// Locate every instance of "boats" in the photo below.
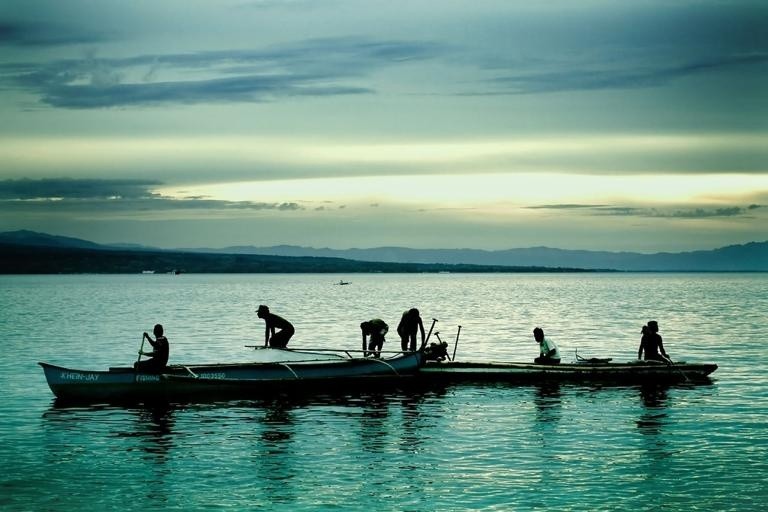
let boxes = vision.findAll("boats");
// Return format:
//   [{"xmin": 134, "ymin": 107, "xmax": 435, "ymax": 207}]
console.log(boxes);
[
  {"xmin": 335, "ymin": 279, "xmax": 353, "ymax": 287},
  {"xmin": 28, "ymin": 348, "xmax": 433, "ymax": 400},
  {"xmin": 422, "ymin": 359, "xmax": 722, "ymax": 385}
]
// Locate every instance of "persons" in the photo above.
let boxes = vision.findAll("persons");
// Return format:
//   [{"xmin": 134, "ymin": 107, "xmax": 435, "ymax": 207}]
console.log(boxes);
[
  {"xmin": 134, "ymin": 323, "xmax": 169, "ymax": 372},
  {"xmin": 396, "ymin": 307, "xmax": 425, "ymax": 353},
  {"xmin": 533, "ymin": 327, "xmax": 562, "ymax": 363},
  {"xmin": 254, "ymin": 304, "xmax": 295, "ymax": 348},
  {"xmin": 638, "ymin": 320, "xmax": 669, "ymax": 362},
  {"xmin": 360, "ymin": 318, "xmax": 389, "ymax": 358}
]
